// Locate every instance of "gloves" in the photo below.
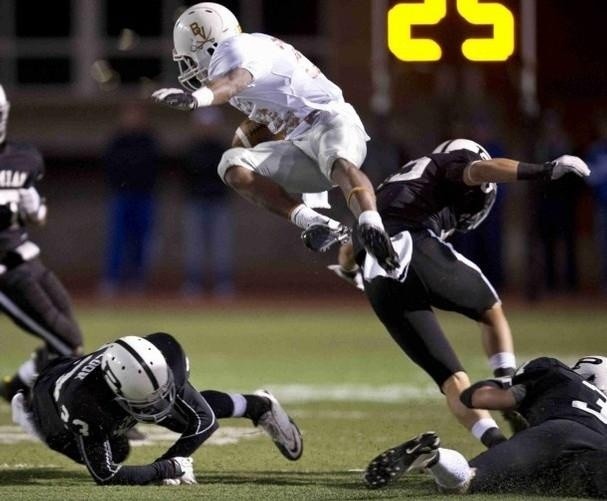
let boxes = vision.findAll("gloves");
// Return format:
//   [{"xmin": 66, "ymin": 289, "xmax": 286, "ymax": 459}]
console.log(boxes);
[
  {"xmin": 327, "ymin": 265, "xmax": 367, "ymax": 291},
  {"xmin": 16, "ymin": 186, "xmax": 49, "ymax": 225},
  {"xmin": 149, "ymin": 86, "xmax": 215, "ymax": 112},
  {"xmin": 516, "ymin": 154, "xmax": 592, "ymax": 186}
]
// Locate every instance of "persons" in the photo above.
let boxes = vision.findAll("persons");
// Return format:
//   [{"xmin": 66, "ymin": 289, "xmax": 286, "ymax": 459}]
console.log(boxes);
[
  {"xmin": 327, "ymin": 139, "xmax": 591, "ymax": 448},
  {"xmin": 364, "ymin": 357, "xmax": 607, "ymax": 500},
  {"xmin": 523, "ymin": 109, "xmax": 580, "ymax": 292},
  {"xmin": 12, "ymin": 333, "xmax": 302, "ymax": 484},
  {"xmin": 103, "ymin": 97, "xmax": 175, "ymax": 296},
  {"xmin": 173, "ymin": 106, "xmax": 238, "ymax": 299},
  {"xmin": 0, "ymin": 84, "xmax": 82, "ymax": 403},
  {"xmin": 154, "ymin": 3, "xmax": 400, "ymax": 278}
]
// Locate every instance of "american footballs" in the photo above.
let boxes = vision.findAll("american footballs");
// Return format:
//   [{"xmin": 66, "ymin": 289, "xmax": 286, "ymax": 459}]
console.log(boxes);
[{"xmin": 232, "ymin": 119, "xmax": 285, "ymax": 149}]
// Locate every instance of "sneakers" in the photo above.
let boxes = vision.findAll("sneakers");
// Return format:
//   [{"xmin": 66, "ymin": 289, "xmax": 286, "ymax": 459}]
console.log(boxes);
[
  {"xmin": 357, "ymin": 224, "xmax": 401, "ymax": 276},
  {"xmin": 249, "ymin": 389, "xmax": 304, "ymax": 463},
  {"xmin": 300, "ymin": 222, "xmax": 354, "ymax": 254},
  {"xmin": 362, "ymin": 431, "xmax": 441, "ymax": 490}
]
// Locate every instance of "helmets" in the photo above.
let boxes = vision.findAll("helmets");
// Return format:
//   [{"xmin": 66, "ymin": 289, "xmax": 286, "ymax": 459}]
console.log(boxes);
[
  {"xmin": 570, "ymin": 357, "xmax": 607, "ymax": 397},
  {"xmin": 172, "ymin": 2, "xmax": 240, "ymax": 92},
  {"xmin": 100, "ymin": 334, "xmax": 176, "ymax": 426},
  {"xmin": 431, "ymin": 138, "xmax": 494, "ymax": 160}
]
